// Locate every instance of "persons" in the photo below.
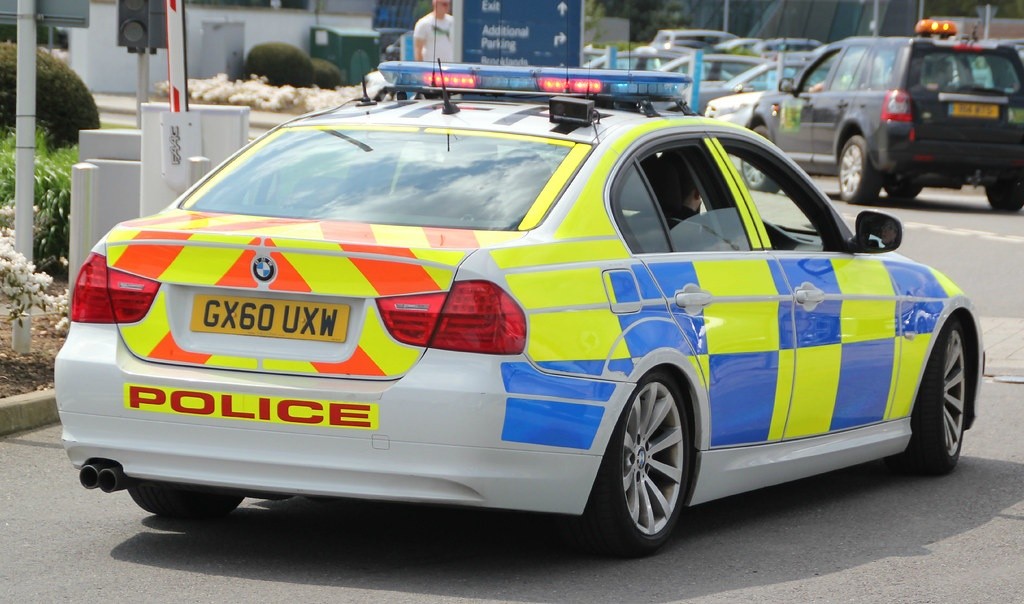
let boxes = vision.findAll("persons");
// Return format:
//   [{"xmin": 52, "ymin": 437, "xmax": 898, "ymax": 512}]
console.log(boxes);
[
  {"xmin": 880, "ymin": 220, "xmax": 899, "ymax": 247},
  {"xmin": 414, "ymin": 0, "xmax": 455, "ymax": 63},
  {"xmin": 652, "ymin": 151, "xmax": 712, "ymax": 231},
  {"xmin": 808, "ymin": 80, "xmax": 826, "ymax": 92}
]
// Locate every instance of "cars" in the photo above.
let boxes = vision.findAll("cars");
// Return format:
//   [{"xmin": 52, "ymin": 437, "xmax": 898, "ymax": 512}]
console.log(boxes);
[
  {"xmin": 580, "ymin": 26, "xmax": 1024, "ymax": 129},
  {"xmin": 51, "ymin": 57, "xmax": 988, "ymax": 560}
]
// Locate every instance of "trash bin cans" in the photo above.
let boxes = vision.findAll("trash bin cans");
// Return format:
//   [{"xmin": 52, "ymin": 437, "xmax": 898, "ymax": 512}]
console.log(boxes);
[
  {"xmin": 310, "ymin": 25, "xmax": 383, "ymax": 88},
  {"xmin": 372, "ymin": 26, "xmax": 408, "ymax": 65}
]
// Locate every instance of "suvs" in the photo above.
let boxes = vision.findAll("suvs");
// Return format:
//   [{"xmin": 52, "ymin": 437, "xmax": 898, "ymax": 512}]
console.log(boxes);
[{"xmin": 741, "ymin": 18, "xmax": 1024, "ymax": 213}]
